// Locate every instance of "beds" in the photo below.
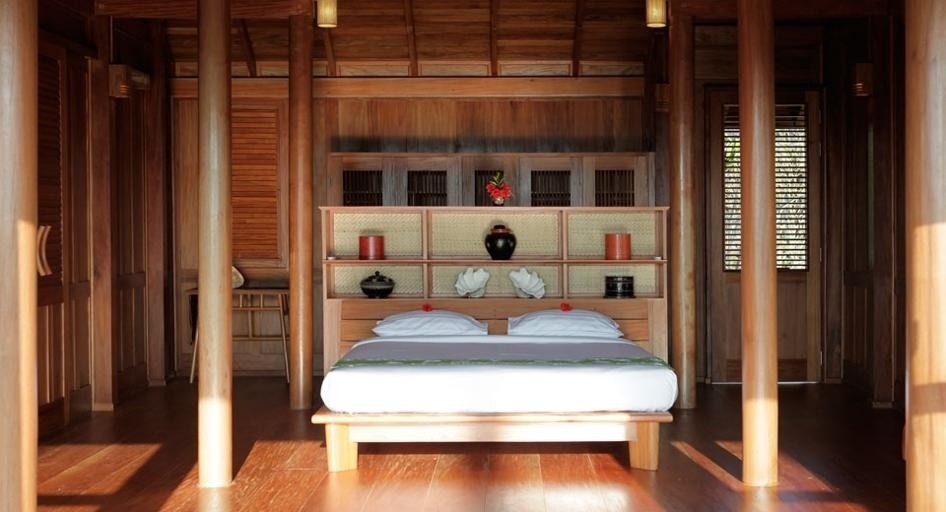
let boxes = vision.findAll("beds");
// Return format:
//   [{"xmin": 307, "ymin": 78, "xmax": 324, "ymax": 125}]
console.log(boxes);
[{"xmin": 311, "ymin": 299, "xmax": 678, "ymax": 472}]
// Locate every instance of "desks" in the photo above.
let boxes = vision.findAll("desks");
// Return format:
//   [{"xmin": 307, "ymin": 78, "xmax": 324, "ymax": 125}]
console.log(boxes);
[{"xmin": 184, "ymin": 287, "xmax": 290, "ymax": 385}]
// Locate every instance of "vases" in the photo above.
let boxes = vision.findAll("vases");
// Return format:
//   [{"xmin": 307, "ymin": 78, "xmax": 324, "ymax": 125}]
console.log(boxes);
[{"xmin": 493, "ymin": 197, "xmax": 505, "ymax": 206}]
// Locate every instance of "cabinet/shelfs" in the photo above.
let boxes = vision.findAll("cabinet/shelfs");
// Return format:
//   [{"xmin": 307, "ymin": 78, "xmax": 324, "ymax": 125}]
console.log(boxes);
[
  {"xmin": 317, "ymin": 205, "xmax": 671, "ymax": 378},
  {"xmin": 326, "ymin": 152, "xmax": 655, "ymax": 206}
]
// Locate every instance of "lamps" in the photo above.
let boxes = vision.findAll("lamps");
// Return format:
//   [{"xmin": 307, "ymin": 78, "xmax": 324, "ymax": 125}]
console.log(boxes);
[
  {"xmin": 850, "ymin": 63, "xmax": 875, "ymax": 97},
  {"xmin": 317, "ymin": 0, "xmax": 338, "ymax": 28},
  {"xmin": 645, "ymin": 0, "xmax": 666, "ymax": 28},
  {"xmin": 655, "ymin": 31, "xmax": 671, "ymax": 112},
  {"xmin": 108, "ymin": 64, "xmax": 132, "ymax": 98}
]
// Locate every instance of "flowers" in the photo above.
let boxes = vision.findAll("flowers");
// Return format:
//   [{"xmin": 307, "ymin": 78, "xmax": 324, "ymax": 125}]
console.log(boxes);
[{"xmin": 486, "ymin": 172, "xmax": 512, "ymax": 200}]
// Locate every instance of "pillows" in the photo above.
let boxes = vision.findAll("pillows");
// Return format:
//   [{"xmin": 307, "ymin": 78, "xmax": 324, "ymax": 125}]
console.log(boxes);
[
  {"xmin": 371, "ymin": 304, "xmax": 488, "ymax": 337},
  {"xmin": 507, "ymin": 303, "xmax": 624, "ymax": 338}
]
